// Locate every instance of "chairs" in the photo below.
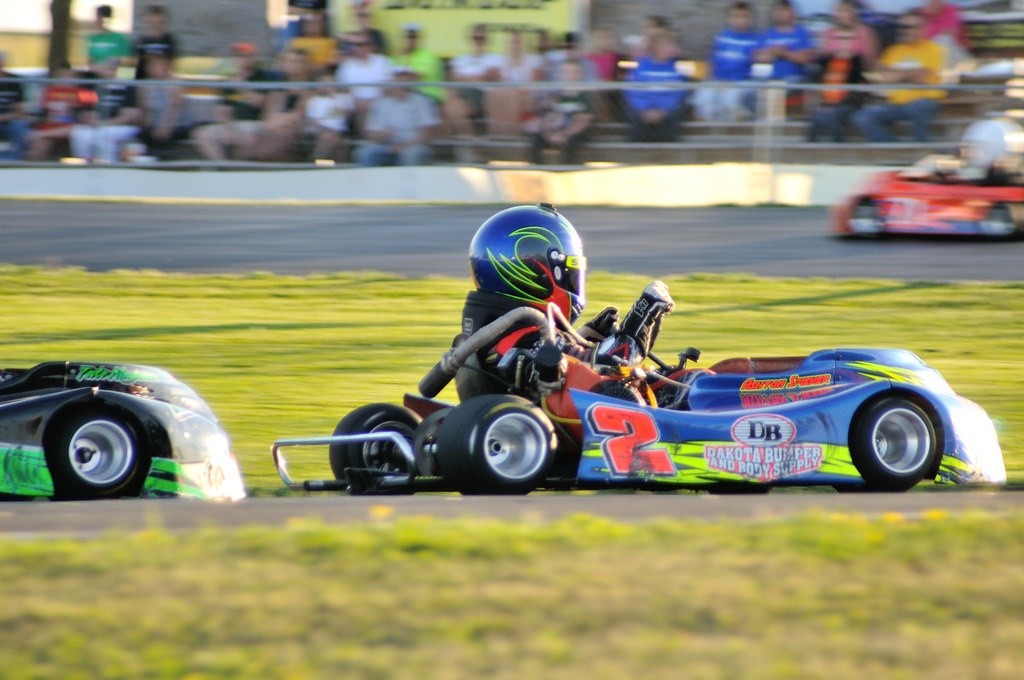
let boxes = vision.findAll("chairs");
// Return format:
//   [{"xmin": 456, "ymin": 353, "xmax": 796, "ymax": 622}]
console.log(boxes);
[{"xmin": 451, "ymin": 334, "xmax": 582, "ymax": 471}]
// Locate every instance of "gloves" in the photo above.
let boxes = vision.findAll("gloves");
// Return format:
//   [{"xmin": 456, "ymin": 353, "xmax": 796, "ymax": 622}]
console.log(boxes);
[
  {"xmin": 587, "ymin": 308, "xmax": 617, "ymax": 342},
  {"xmin": 645, "ymin": 281, "xmax": 674, "ymax": 314}
]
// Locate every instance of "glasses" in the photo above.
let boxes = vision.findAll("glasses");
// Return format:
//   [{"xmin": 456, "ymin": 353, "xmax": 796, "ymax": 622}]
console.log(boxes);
[{"xmin": 348, "ymin": 41, "xmax": 373, "ymax": 48}]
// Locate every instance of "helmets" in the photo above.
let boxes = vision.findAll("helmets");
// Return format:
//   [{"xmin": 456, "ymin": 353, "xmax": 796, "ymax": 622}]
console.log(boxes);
[
  {"xmin": 961, "ymin": 118, "xmax": 1024, "ymax": 185},
  {"xmin": 469, "ymin": 204, "xmax": 585, "ymax": 332}
]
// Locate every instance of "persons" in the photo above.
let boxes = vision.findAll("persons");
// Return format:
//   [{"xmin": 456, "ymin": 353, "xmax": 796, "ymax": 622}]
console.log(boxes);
[
  {"xmin": 938, "ymin": 117, "xmax": 1024, "ymax": 186},
  {"xmin": 460, "ymin": 200, "xmax": 676, "ymax": 403},
  {"xmin": 0, "ymin": 0, "xmax": 973, "ymax": 165}
]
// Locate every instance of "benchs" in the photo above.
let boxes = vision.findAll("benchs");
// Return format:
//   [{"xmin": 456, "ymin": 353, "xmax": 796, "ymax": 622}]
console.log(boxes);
[{"xmin": 179, "ymin": 71, "xmax": 1024, "ymax": 152}]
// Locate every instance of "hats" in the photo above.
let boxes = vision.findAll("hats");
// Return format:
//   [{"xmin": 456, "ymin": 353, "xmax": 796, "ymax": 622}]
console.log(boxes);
[
  {"xmin": 391, "ymin": 66, "xmax": 421, "ymax": 79},
  {"xmin": 230, "ymin": 42, "xmax": 256, "ymax": 58}
]
